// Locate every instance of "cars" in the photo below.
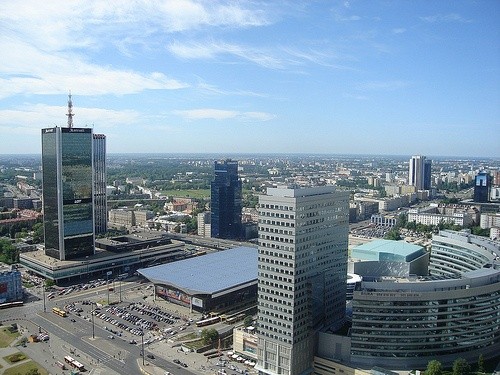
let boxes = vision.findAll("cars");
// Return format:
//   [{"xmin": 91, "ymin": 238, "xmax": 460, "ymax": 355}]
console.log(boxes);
[
  {"xmin": 47, "ymin": 272, "xmax": 137, "ymax": 300},
  {"xmin": 180, "ymin": 362, "xmax": 187, "ymax": 367},
  {"xmin": 174, "ymin": 359, "xmax": 180, "ymax": 363},
  {"xmin": 92, "ymin": 302, "xmax": 193, "ymax": 346},
  {"xmin": 39, "ymin": 333, "xmax": 49, "ymax": 341},
  {"xmin": 148, "ymin": 354, "xmax": 154, "ymax": 359}
]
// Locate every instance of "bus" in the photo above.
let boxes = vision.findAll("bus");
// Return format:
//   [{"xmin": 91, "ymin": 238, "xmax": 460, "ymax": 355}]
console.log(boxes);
[
  {"xmin": 196, "ymin": 316, "xmax": 220, "ymax": 327},
  {"xmin": 53, "ymin": 306, "xmax": 66, "ymax": 317},
  {"xmin": 64, "ymin": 355, "xmax": 84, "ymax": 371},
  {"xmin": 226, "ymin": 313, "xmax": 246, "ymax": 325}
]
